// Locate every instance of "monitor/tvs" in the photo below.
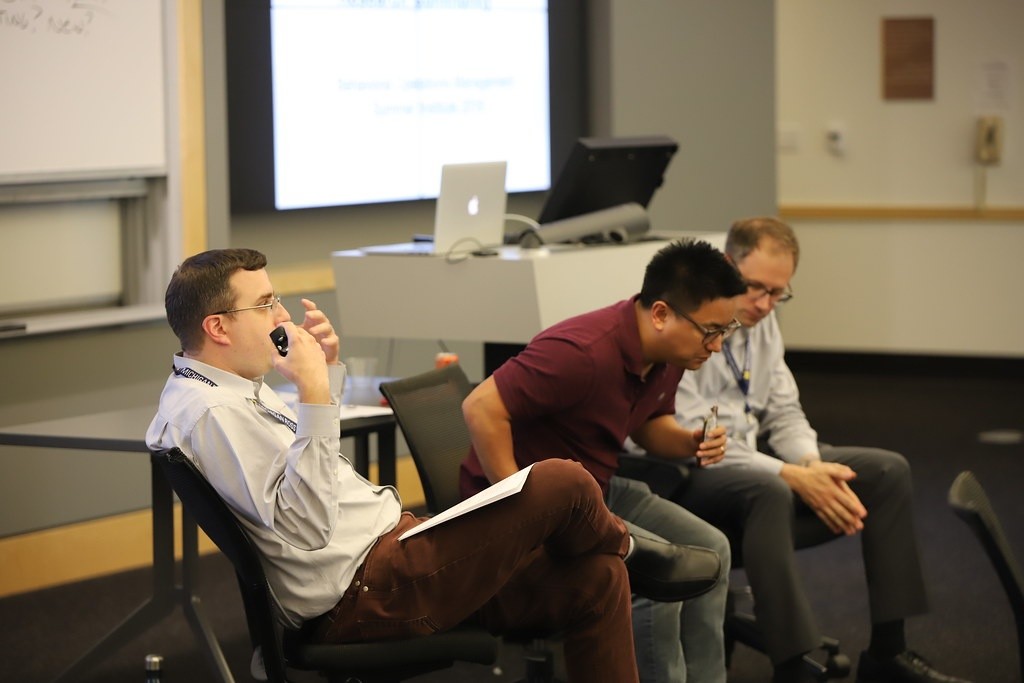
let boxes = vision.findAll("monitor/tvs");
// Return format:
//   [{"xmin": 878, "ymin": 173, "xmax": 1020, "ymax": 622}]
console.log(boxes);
[{"xmin": 536, "ymin": 134, "xmax": 678, "ymax": 225}]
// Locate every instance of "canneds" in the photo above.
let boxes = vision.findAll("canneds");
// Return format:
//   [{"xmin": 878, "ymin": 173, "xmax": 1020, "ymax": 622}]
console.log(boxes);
[{"xmin": 435, "ymin": 352, "xmax": 459, "ymax": 368}]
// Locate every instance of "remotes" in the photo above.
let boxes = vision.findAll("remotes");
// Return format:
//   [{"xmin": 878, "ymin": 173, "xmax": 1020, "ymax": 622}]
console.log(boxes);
[{"xmin": 269, "ymin": 325, "xmax": 289, "ymax": 357}]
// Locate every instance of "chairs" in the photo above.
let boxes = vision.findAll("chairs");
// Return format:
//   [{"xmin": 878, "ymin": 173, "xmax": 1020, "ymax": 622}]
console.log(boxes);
[
  {"xmin": 149, "ymin": 447, "xmax": 501, "ymax": 683},
  {"xmin": 378, "ymin": 366, "xmax": 850, "ymax": 683},
  {"xmin": 948, "ymin": 470, "xmax": 1024, "ymax": 677}
]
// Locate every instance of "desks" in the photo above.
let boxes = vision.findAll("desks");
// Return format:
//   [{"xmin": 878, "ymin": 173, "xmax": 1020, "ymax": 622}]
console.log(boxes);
[
  {"xmin": 0, "ymin": 392, "xmax": 399, "ymax": 683},
  {"xmin": 333, "ymin": 232, "xmax": 729, "ymax": 380}
]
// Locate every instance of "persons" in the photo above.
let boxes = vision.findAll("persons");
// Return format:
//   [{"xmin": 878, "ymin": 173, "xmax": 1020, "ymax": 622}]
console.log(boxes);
[
  {"xmin": 142, "ymin": 248, "xmax": 721, "ymax": 683},
  {"xmin": 625, "ymin": 218, "xmax": 968, "ymax": 683},
  {"xmin": 456, "ymin": 239, "xmax": 749, "ymax": 683}
]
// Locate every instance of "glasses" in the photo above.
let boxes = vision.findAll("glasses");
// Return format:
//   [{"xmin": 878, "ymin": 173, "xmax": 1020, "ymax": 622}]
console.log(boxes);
[
  {"xmin": 729, "ymin": 254, "xmax": 793, "ymax": 302},
  {"xmin": 673, "ymin": 303, "xmax": 742, "ymax": 347},
  {"xmin": 210, "ymin": 293, "xmax": 280, "ymax": 314}
]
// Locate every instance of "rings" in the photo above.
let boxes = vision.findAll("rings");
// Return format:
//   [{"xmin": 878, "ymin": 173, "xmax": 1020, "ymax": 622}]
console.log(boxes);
[{"xmin": 721, "ymin": 446, "xmax": 725, "ymax": 453}]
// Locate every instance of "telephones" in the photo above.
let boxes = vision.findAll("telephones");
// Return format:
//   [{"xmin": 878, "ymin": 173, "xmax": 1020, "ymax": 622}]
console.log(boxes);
[{"xmin": 975, "ymin": 117, "xmax": 1003, "ymax": 163}]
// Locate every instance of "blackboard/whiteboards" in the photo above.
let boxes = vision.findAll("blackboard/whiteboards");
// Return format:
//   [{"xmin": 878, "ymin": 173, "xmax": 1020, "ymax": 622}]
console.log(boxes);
[{"xmin": 0, "ymin": 0, "xmax": 168, "ymax": 184}]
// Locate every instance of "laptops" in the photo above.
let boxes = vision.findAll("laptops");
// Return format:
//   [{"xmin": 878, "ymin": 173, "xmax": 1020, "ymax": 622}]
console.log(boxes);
[{"xmin": 363, "ymin": 160, "xmax": 507, "ymax": 257}]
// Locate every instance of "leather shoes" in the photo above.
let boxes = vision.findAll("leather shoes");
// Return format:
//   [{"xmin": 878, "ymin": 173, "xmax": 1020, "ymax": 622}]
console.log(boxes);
[
  {"xmin": 856, "ymin": 646, "xmax": 967, "ymax": 683},
  {"xmin": 626, "ymin": 531, "xmax": 722, "ymax": 604},
  {"xmin": 772, "ymin": 654, "xmax": 828, "ymax": 683}
]
